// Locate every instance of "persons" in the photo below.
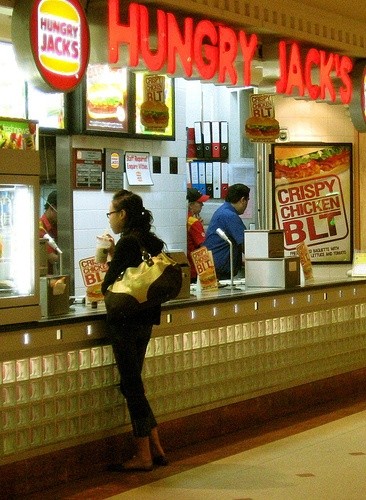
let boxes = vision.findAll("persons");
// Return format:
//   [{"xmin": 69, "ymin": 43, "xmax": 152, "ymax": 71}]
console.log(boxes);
[
  {"xmin": 187, "ymin": 187, "xmax": 209, "ymax": 282},
  {"xmin": 201, "ymin": 183, "xmax": 250, "ymax": 280},
  {"xmin": 100, "ymin": 190, "xmax": 181, "ymax": 471},
  {"xmin": 39, "ymin": 191, "xmax": 57, "ymax": 273}
]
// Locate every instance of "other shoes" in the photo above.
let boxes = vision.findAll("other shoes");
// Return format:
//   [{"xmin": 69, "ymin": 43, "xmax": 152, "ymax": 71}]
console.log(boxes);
[{"xmin": 108, "ymin": 455, "xmax": 169, "ymax": 473}]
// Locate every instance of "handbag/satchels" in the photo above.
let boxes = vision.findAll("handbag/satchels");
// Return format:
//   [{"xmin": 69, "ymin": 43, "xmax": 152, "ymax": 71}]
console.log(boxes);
[{"xmin": 104, "ymin": 234, "xmax": 183, "ymax": 324}]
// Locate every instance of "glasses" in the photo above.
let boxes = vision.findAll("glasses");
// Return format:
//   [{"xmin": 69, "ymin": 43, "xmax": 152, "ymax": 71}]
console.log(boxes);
[{"xmin": 106, "ymin": 210, "xmax": 119, "ymax": 218}]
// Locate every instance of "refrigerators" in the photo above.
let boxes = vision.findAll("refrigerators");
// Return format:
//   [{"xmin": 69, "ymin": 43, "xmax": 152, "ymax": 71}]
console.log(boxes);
[{"xmin": 2, "ymin": 113, "xmax": 41, "ymax": 327}]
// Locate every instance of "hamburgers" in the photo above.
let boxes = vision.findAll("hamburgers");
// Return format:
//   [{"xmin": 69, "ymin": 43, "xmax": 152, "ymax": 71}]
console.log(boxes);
[
  {"xmin": 275, "ymin": 144, "xmax": 350, "ymax": 184},
  {"xmin": 88, "ymin": 88, "xmax": 168, "ymax": 130},
  {"xmin": 87, "ymin": 284, "xmax": 102, "ymax": 301},
  {"xmin": 200, "ymin": 270, "xmax": 216, "ymax": 288},
  {"xmin": 245, "ymin": 117, "xmax": 280, "ymax": 142}
]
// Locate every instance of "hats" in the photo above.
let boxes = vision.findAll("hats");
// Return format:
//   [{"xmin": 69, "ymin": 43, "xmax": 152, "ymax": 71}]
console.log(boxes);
[{"xmin": 186, "ymin": 187, "xmax": 210, "ymax": 203}]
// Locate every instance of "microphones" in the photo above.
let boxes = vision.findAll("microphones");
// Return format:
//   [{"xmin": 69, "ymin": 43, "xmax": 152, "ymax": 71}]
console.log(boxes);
[
  {"xmin": 42, "ymin": 234, "xmax": 62, "ymax": 275},
  {"xmin": 216, "ymin": 227, "xmax": 241, "ymax": 290}
]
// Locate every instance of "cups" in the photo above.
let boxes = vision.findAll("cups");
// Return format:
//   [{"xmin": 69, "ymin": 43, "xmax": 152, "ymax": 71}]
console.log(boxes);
[{"xmin": 94, "ymin": 235, "xmax": 112, "ymax": 264}]
